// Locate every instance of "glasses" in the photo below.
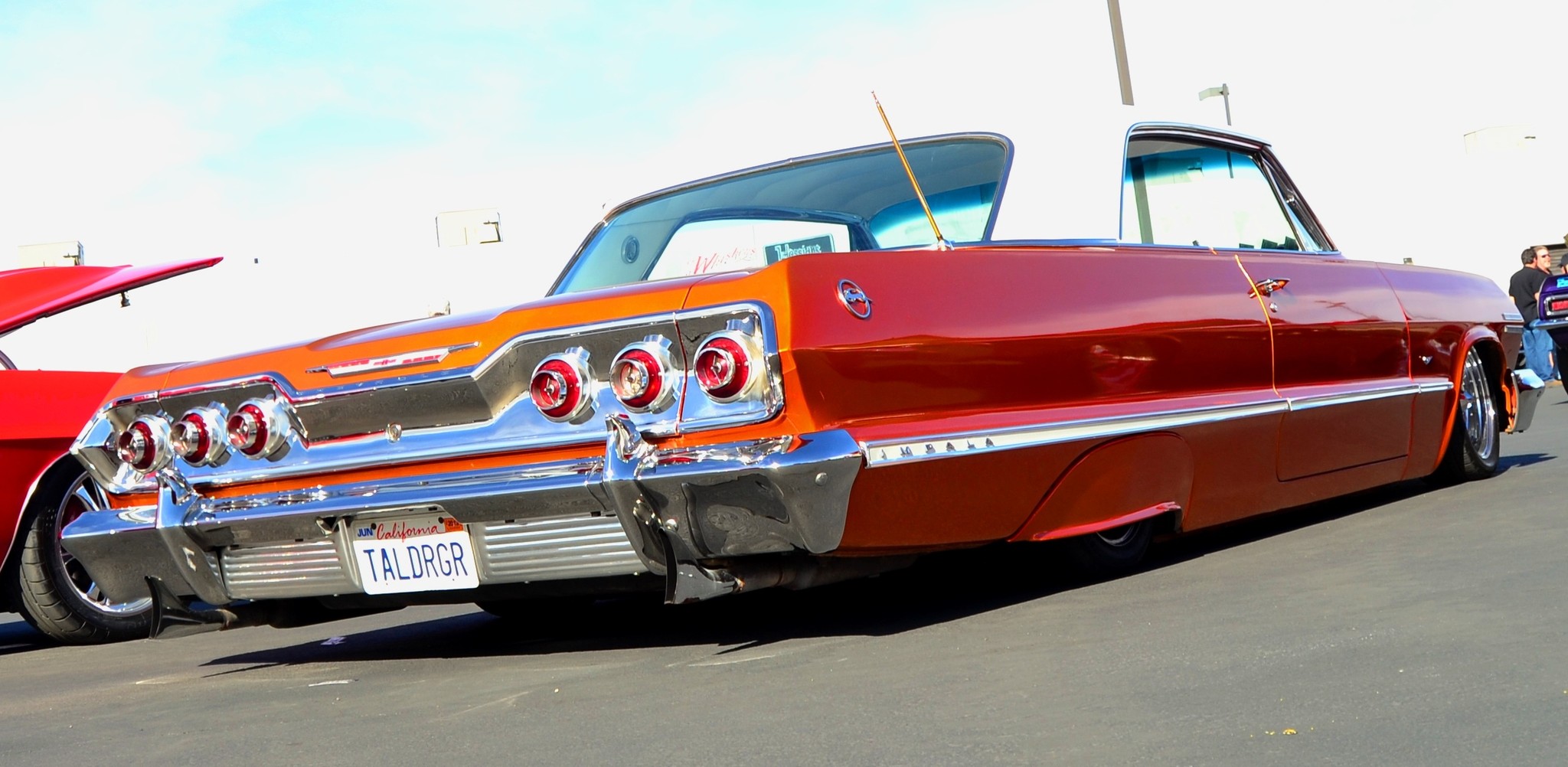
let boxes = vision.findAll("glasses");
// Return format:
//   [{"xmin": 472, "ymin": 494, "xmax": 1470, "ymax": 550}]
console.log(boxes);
[
  {"xmin": 1560, "ymin": 265, "xmax": 1563, "ymax": 268},
  {"xmin": 1542, "ymin": 254, "xmax": 1550, "ymax": 258}
]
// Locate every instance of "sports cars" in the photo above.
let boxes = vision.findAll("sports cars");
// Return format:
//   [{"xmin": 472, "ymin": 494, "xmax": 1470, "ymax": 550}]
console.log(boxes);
[
  {"xmin": 63, "ymin": 89, "xmax": 1546, "ymax": 632},
  {"xmin": 0, "ymin": 257, "xmax": 228, "ymax": 650}
]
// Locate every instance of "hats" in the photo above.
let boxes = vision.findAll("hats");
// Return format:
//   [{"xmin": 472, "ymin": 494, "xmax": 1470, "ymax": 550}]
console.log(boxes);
[{"xmin": 1557, "ymin": 253, "xmax": 1568, "ymax": 266}]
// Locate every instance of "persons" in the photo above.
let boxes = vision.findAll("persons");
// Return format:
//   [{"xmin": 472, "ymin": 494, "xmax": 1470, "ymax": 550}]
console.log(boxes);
[
  {"xmin": 1508, "ymin": 248, "xmax": 1565, "ymax": 387},
  {"xmin": 1558, "ymin": 251, "xmax": 1568, "ymax": 282},
  {"xmin": 1529, "ymin": 245, "xmax": 1560, "ymax": 381}
]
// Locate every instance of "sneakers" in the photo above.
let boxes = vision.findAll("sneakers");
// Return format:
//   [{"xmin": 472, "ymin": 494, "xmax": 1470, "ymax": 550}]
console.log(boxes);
[{"xmin": 1545, "ymin": 379, "xmax": 1562, "ymax": 387}]
